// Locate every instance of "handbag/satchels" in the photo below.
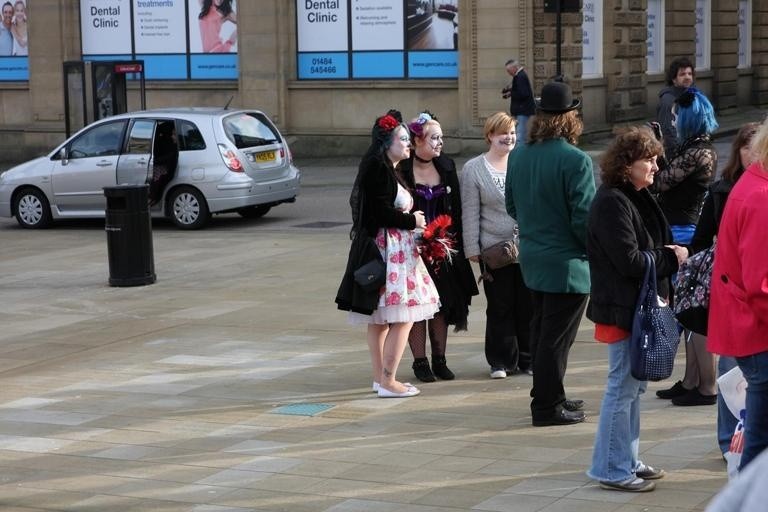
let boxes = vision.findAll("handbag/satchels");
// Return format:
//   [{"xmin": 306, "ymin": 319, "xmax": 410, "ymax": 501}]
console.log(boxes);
[{"xmin": 629, "ymin": 249, "xmax": 680, "ymax": 382}]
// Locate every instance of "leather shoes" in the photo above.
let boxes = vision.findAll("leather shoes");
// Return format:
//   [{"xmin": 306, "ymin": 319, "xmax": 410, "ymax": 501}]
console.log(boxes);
[
  {"xmin": 373, "ymin": 381, "xmax": 415, "ymax": 394},
  {"xmin": 532, "ymin": 406, "xmax": 586, "ymax": 427},
  {"xmin": 562, "ymin": 400, "xmax": 586, "ymax": 411},
  {"xmin": 672, "ymin": 386, "xmax": 718, "ymax": 407},
  {"xmin": 655, "ymin": 379, "xmax": 693, "ymax": 400},
  {"xmin": 378, "ymin": 387, "xmax": 421, "ymax": 399}
]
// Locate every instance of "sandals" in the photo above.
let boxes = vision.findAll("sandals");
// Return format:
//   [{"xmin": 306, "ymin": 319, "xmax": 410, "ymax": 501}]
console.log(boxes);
[
  {"xmin": 636, "ymin": 462, "xmax": 666, "ymax": 480},
  {"xmin": 600, "ymin": 473, "xmax": 656, "ymax": 492}
]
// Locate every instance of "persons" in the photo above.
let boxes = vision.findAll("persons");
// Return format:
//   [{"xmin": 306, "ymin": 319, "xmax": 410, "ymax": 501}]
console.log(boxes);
[
  {"xmin": 708, "ymin": 117, "xmax": 766, "ymax": 476},
  {"xmin": 335, "ymin": 110, "xmax": 442, "ymax": 398},
  {"xmin": 147, "ymin": 130, "xmax": 184, "ymax": 205},
  {"xmin": 9, "ymin": 1, "xmax": 27, "ymax": 56},
  {"xmin": 658, "ymin": 60, "xmax": 702, "ymax": 151},
  {"xmin": 651, "ymin": 94, "xmax": 718, "ymax": 406},
  {"xmin": 198, "ymin": 0, "xmax": 236, "ymax": 53},
  {"xmin": 0, "ymin": 2, "xmax": 13, "ymax": 56},
  {"xmin": 587, "ymin": 124, "xmax": 688, "ymax": 494},
  {"xmin": 504, "ymin": 60, "xmax": 537, "ymax": 146},
  {"xmin": 504, "ymin": 82, "xmax": 596, "ymax": 425},
  {"xmin": 459, "ymin": 114, "xmax": 532, "ymax": 377},
  {"xmin": 666, "ymin": 123, "xmax": 759, "ymax": 461},
  {"xmin": 397, "ymin": 112, "xmax": 479, "ymax": 381}
]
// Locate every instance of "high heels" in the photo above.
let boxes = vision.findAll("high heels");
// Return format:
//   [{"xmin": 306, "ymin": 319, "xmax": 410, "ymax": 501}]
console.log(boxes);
[
  {"xmin": 412, "ymin": 356, "xmax": 437, "ymax": 383},
  {"xmin": 430, "ymin": 354, "xmax": 456, "ymax": 381}
]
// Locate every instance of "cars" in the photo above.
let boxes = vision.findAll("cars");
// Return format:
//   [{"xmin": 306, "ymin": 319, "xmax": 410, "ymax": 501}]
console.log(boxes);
[{"xmin": 0, "ymin": 96, "xmax": 301, "ymax": 229}]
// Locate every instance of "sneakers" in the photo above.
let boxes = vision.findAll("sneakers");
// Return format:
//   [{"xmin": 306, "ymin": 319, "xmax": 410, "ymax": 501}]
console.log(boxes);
[
  {"xmin": 524, "ymin": 364, "xmax": 534, "ymax": 375},
  {"xmin": 489, "ymin": 365, "xmax": 508, "ymax": 379}
]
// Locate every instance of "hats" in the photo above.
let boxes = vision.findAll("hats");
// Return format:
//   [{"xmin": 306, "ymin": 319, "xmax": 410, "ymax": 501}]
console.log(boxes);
[{"xmin": 535, "ymin": 83, "xmax": 581, "ymax": 115}]
